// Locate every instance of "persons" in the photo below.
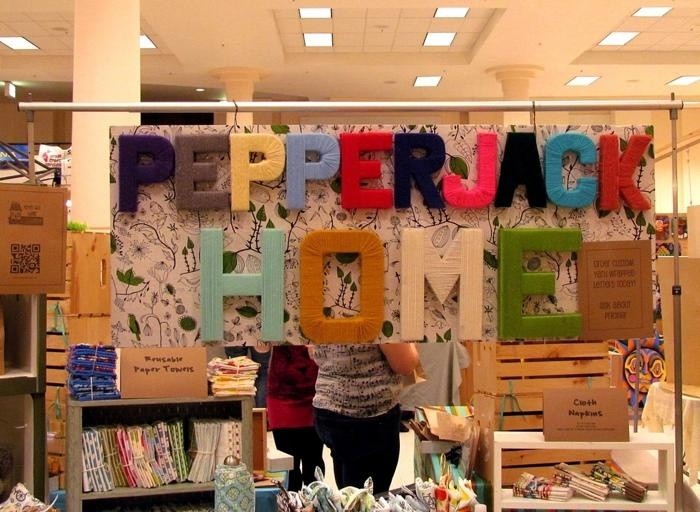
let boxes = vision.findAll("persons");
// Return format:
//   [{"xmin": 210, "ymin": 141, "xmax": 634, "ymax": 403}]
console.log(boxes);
[
  {"xmin": 305, "ymin": 343, "xmax": 420, "ymax": 494},
  {"xmin": 266, "ymin": 342, "xmax": 325, "ymax": 493}
]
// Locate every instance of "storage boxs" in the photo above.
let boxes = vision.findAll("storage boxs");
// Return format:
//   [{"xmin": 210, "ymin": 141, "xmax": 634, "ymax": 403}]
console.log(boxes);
[
  {"xmin": 472, "ymin": 392, "xmax": 613, "ymax": 490},
  {"xmin": 472, "ymin": 339, "xmax": 611, "ymax": 397},
  {"xmin": 41, "ymin": 227, "xmax": 117, "ymax": 491}
]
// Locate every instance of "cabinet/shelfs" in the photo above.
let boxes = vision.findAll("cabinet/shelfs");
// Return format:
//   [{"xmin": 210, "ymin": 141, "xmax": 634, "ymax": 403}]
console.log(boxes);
[
  {"xmin": 0, "ymin": 293, "xmax": 45, "ymax": 512},
  {"xmin": 65, "ymin": 395, "xmax": 254, "ymax": 512},
  {"xmin": 494, "ymin": 432, "xmax": 674, "ymax": 512}
]
